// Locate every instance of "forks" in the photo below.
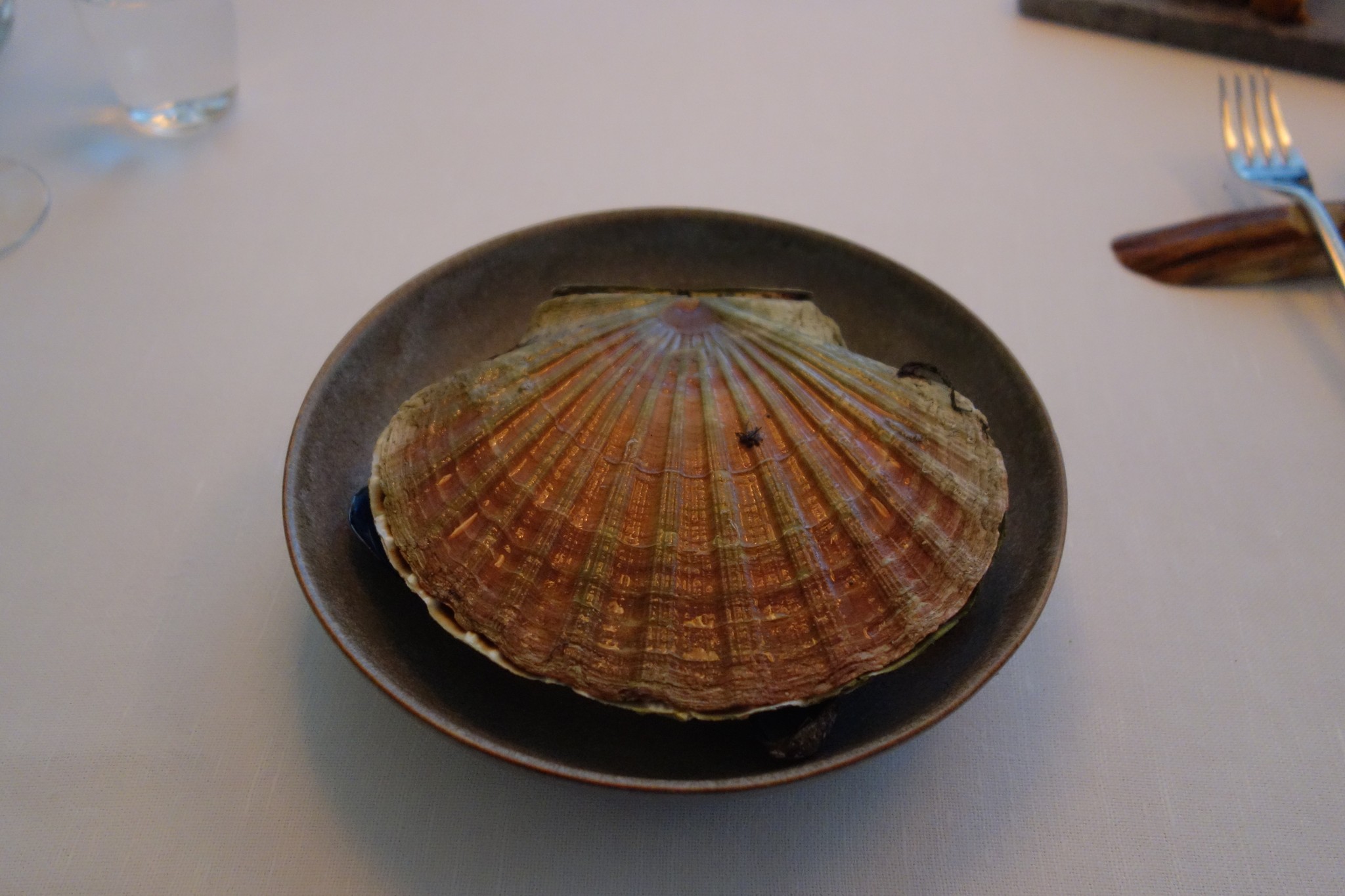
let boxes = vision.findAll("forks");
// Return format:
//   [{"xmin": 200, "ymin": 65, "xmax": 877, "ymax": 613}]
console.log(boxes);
[{"xmin": 1218, "ymin": 72, "xmax": 1345, "ymax": 296}]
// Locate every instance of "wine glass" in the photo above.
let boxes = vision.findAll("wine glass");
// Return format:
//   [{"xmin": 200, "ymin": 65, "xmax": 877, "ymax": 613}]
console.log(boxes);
[{"xmin": 2, "ymin": 0, "xmax": 49, "ymax": 256}]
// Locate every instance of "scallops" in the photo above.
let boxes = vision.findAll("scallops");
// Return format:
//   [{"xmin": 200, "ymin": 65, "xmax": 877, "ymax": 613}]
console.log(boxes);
[{"xmin": 370, "ymin": 293, "xmax": 1009, "ymax": 722}]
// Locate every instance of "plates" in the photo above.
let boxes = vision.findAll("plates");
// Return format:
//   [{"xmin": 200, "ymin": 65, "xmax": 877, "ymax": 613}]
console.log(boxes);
[{"xmin": 283, "ymin": 205, "xmax": 1068, "ymax": 792}]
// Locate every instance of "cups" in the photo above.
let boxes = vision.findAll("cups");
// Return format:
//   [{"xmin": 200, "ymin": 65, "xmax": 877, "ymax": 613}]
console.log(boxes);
[{"xmin": 75, "ymin": 0, "xmax": 239, "ymax": 135}]
